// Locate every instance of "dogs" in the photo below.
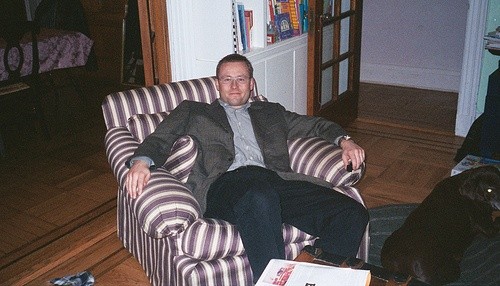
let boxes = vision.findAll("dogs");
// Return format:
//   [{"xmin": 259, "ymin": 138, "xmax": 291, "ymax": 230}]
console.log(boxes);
[{"xmin": 379, "ymin": 163, "xmax": 500, "ymax": 286}]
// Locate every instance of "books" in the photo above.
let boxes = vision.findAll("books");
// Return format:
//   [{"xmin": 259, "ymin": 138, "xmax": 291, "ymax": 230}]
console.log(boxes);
[
  {"xmin": 232, "ymin": 0, "xmax": 254, "ymax": 55},
  {"xmin": 451, "ymin": 154, "xmax": 500, "ymax": 177},
  {"xmin": 255, "ymin": 259, "xmax": 371, "ymax": 286}
]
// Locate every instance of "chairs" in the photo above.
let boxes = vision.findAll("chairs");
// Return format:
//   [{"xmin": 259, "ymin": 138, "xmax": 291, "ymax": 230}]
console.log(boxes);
[{"xmin": 0, "ymin": 0, "xmax": 89, "ymax": 159}]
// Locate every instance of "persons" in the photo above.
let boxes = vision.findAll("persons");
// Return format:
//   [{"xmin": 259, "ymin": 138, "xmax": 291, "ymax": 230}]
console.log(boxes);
[{"xmin": 124, "ymin": 54, "xmax": 370, "ymax": 284}]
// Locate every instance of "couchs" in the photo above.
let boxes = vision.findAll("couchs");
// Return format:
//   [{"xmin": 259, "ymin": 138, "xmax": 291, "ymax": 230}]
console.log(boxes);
[{"xmin": 102, "ymin": 77, "xmax": 369, "ymax": 286}]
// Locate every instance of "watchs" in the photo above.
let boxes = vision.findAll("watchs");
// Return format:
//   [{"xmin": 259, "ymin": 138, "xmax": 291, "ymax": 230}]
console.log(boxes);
[{"xmin": 338, "ymin": 134, "xmax": 352, "ymax": 148}]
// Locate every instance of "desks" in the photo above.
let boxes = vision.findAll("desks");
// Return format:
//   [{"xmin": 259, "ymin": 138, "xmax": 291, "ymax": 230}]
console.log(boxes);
[{"xmin": 0, "ymin": 28, "xmax": 95, "ymax": 80}]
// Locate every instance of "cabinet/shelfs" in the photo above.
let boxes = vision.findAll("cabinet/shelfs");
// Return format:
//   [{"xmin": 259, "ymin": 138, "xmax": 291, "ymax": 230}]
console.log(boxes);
[{"xmin": 252, "ymin": 34, "xmax": 333, "ymax": 116}]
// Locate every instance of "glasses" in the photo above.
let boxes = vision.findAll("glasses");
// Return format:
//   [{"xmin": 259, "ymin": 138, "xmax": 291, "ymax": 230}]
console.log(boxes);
[{"xmin": 219, "ymin": 77, "xmax": 247, "ymax": 84}]
